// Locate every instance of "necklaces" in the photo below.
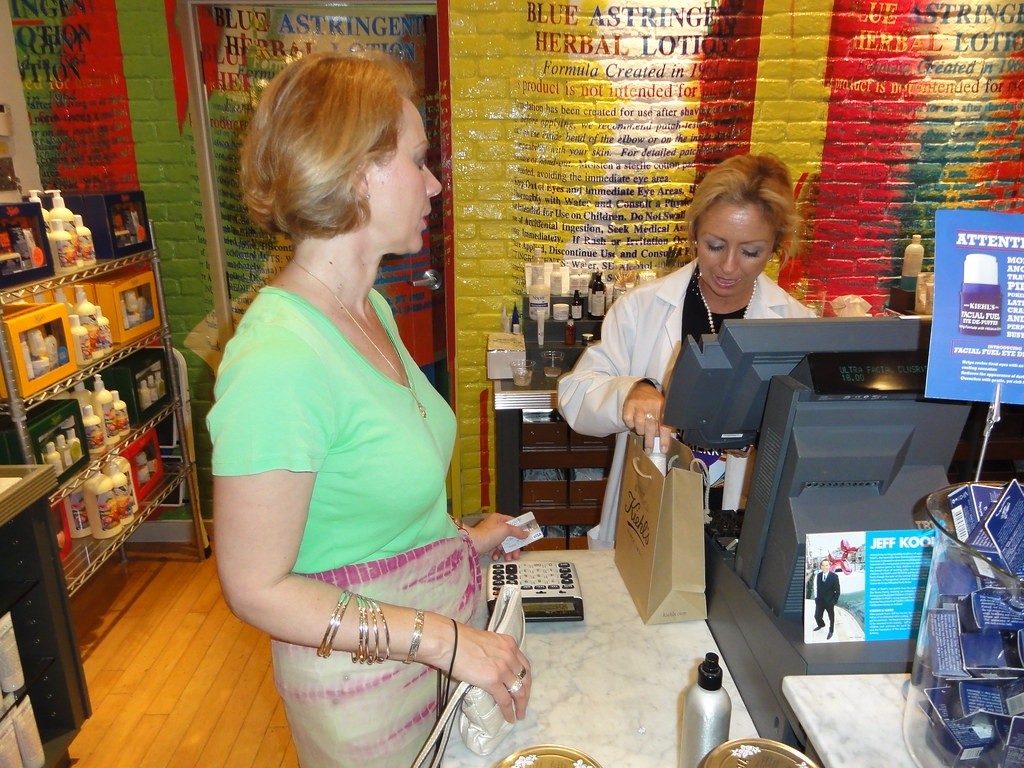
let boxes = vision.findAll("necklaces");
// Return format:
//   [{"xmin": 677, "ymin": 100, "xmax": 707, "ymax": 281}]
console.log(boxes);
[
  {"xmin": 294, "ymin": 255, "xmax": 428, "ymax": 418},
  {"xmin": 696, "ymin": 274, "xmax": 758, "ymax": 335}
]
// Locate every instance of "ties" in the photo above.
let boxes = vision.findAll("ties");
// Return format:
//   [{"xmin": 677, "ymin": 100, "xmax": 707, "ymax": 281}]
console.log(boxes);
[{"xmin": 824, "ymin": 576, "xmax": 826, "ymax": 580}]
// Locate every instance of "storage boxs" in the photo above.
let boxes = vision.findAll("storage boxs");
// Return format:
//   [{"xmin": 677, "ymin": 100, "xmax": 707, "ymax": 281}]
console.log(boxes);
[
  {"xmin": 85, "ymin": 349, "xmax": 173, "ymax": 425},
  {"xmin": 0, "ymin": 301, "xmax": 77, "ymax": 398},
  {"xmin": 95, "ymin": 269, "xmax": 161, "ymax": 344},
  {"xmin": 0, "ymin": 203, "xmax": 54, "ymax": 287},
  {"xmin": 84, "ymin": 192, "xmax": 153, "ymax": 259},
  {"xmin": 487, "ymin": 333, "xmax": 526, "ymax": 379},
  {"xmin": 2, "ymin": 400, "xmax": 91, "ymax": 486},
  {"xmin": 116, "ymin": 428, "xmax": 164, "ymax": 502}
]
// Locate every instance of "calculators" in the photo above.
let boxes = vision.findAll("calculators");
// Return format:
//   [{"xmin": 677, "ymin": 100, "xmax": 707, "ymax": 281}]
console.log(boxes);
[{"xmin": 486, "ymin": 561, "xmax": 583, "ymax": 622}]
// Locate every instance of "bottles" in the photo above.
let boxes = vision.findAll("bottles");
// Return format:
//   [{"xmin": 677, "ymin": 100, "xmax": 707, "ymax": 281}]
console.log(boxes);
[
  {"xmin": 94, "ymin": 305, "xmax": 114, "ymax": 354},
  {"xmin": 47, "ymin": 219, "xmax": 78, "ymax": 274},
  {"xmin": 613, "ymin": 283, "xmax": 626, "ymax": 304},
  {"xmin": 900, "ymin": 234, "xmax": 924, "ymax": 289},
  {"xmin": 532, "ymin": 247, "xmax": 546, "ymax": 286},
  {"xmin": 69, "ymin": 315, "xmax": 93, "ymax": 365},
  {"xmin": 586, "ymin": 270, "xmax": 606, "ymax": 319},
  {"xmin": 43, "ymin": 428, "xmax": 84, "ymax": 476},
  {"xmin": 74, "ymin": 215, "xmax": 96, "ymax": 265},
  {"xmin": 645, "ymin": 436, "xmax": 666, "ymax": 478},
  {"xmin": 554, "ymin": 304, "xmax": 570, "ymax": 322},
  {"xmin": 529, "ymin": 278, "xmax": 550, "ymax": 320},
  {"xmin": 140, "ymin": 371, "xmax": 167, "ymax": 409},
  {"xmin": 119, "ymin": 288, "xmax": 155, "ymax": 329},
  {"xmin": 82, "ymin": 405, "xmax": 106, "ymax": 452},
  {"xmin": 111, "ymin": 390, "xmax": 131, "ymax": 436},
  {"xmin": 679, "ymin": 652, "xmax": 732, "ymax": 768},
  {"xmin": 550, "ymin": 263, "xmax": 562, "ymax": 296},
  {"xmin": 19, "ymin": 322, "xmax": 69, "ymax": 380}
]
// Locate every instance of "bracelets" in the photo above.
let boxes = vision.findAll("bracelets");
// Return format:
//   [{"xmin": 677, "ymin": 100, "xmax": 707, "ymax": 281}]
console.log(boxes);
[{"xmin": 318, "ymin": 589, "xmax": 424, "ymax": 663}]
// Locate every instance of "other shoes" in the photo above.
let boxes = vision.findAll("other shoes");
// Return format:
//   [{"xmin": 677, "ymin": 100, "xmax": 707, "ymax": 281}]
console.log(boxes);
[
  {"xmin": 814, "ymin": 622, "xmax": 826, "ymax": 633},
  {"xmin": 830, "ymin": 629, "xmax": 835, "ymax": 641}
]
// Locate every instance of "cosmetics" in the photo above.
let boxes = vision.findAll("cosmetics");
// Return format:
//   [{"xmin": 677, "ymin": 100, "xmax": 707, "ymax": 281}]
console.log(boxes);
[{"xmin": 0, "ymin": 610, "xmax": 45, "ymax": 767}]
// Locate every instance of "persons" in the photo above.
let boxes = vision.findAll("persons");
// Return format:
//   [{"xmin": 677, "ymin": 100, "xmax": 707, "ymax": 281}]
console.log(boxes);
[
  {"xmin": 558, "ymin": 154, "xmax": 818, "ymax": 549},
  {"xmin": 205, "ymin": 57, "xmax": 532, "ymax": 765},
  {"xmin": 814, "ymin": 560, "xmax": 840, "ymax": 640}
]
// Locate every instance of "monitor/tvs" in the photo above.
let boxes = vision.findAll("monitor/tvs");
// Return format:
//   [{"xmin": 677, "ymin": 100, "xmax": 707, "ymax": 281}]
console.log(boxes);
[{"xmin": 661, "ymin": 312, "xmax": 940, "ymax": 449}]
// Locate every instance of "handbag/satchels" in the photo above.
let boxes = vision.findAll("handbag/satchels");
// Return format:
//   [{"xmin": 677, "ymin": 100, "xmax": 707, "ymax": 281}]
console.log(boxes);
[
  {"xmin": 412, "ymin": 581, "xmax": 528, "ymax": 768},
  {"xmin": 615, "ymin": 438, "xmax": 707, "ymax": 625}
]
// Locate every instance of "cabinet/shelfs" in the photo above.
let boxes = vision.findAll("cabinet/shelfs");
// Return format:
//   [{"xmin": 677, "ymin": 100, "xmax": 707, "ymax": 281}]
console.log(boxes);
[
  {"xmin": 0, "ymin": 466, "xmax": 92, "ymax": 768},
  {"xmin": 0, "ymin": 217, "xmax": 204, "ymax": 583},
  {"xmin": 491, "ymin": 370, "xmax": 619, "ymax": 555}
]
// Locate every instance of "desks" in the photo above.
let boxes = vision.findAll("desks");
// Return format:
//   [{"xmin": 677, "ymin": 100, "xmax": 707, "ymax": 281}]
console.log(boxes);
[{"xmin": 438, "ymin": 549, "xmax": 761, "ymax": 768}]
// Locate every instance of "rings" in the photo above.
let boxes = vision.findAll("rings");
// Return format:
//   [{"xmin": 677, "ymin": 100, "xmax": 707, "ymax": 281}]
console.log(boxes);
[
  {"xmin": 509, "ymin": 677, "xmax": 523, "ymax": 693},
  {"xmin": 519, "ymin": 665, "xmax": 526, "ymax": 678},
  {"xmin": 646, "ymin": 413, "xmax": 656, "ymax": 421}
]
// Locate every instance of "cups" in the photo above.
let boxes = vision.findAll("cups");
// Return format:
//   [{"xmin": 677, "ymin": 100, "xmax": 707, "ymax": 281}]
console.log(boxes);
[
  {"xmin": 789, "ymin": 282, "xmax": 826, "ymax": 318},
  {"xmin": 510, "ymin": 360, "xmax": 535, "ymax": 385},
  {"xmin": 541, "ymin": 350, "xmax": 565, "ymax": 377}
]
